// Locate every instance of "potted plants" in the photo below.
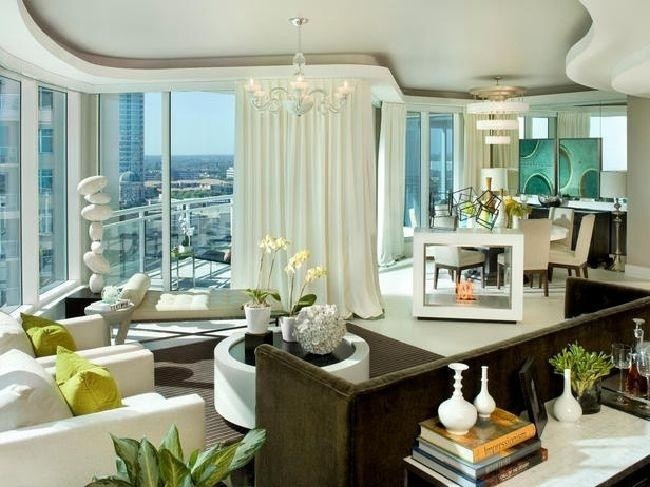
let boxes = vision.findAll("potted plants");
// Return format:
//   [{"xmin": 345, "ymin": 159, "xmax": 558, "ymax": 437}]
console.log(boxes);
[
  {"xmin": 242, "ymin": 234, "xmax": 328, "ymax": 343},
  {"xmin": 461, "ymin": 199, "xmax": 532, "ymax": 230},
  {"xmin": 548, "ymin": 340, "xmax": 616, "ymax": 415}
]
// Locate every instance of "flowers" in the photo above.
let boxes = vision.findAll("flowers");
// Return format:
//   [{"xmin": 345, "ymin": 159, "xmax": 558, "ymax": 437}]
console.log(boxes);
[
  {"xmin": 176, "ymin": 205, "xmax": 193, "ymax": 246},
  {"xmin": 292, "ymin": 303, "xmax": 347, "ymax": 355}
]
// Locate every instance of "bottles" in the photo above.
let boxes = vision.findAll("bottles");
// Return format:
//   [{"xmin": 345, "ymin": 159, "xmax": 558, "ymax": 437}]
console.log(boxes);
[{"xmin": 626, "ymin": 329, "xmax": 649, "ymax": 397}]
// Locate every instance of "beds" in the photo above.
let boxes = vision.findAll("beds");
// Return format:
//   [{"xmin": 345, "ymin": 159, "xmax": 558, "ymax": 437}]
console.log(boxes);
[{"xmin": 120, "ymin": 272, "xmax": 283, "ymax": 320}]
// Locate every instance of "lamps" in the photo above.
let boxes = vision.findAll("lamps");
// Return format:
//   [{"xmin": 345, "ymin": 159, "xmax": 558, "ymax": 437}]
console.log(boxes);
[
  {"xmin": 599, "ymin": 170, "xmax": 628, "ymax": 271},
  {"xmin": 465, "ymin": 76, "xmax": 529, "ymax": 192},
  {"xmin": 244, "ymin": 17, "xmax": 356, "ymax": 116}
]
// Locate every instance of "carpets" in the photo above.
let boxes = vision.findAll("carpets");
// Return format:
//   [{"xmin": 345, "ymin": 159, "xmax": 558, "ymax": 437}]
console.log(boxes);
[{"xmin": 139, "ymin": 324, "xmax": 447, "ymax": 487}]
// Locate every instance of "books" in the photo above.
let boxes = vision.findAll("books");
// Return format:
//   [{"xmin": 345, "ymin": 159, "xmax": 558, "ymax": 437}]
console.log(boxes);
[{"xmin": 411, "ymin": 407, "xmax": 548, "ymax": 487}]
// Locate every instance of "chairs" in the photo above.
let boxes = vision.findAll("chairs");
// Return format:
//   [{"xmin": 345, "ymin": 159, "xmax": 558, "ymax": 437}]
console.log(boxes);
[
  {"xmin": 432, "ymin": 202, "xmax": 596, "ymax": 297},
  {"xmin": 192, "ymin": 246, "xmax": 231, "ymax": 287}
]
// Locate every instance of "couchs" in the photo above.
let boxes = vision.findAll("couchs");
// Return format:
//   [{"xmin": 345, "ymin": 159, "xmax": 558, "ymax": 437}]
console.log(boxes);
[
  {"xmin": 254, "ymin": 277, "xmax": 650, "ymax": 486},
  {"xmin": 0, "ymin": 310, "xmax": 207, "ymax": 487}
]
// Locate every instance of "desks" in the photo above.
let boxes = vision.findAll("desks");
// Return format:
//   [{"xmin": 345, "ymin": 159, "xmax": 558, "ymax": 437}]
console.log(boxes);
[
  {"xmin": 64, "ymin": 288, "xmax": 135, "ymax": 346},
  {"xmin": 411, "ymin": 210, "xmax": 569, "ymax": 322},
  {"xmin": 213, "ymin": 327, "xmax": 370, "ymax": 431},
  {"xmin": 402, "ymin": 370, "xmax": 646, "ymax": 487},
  {"xmin": 171, "ymin": 252, "xmax": 196, "ymax": 291}
]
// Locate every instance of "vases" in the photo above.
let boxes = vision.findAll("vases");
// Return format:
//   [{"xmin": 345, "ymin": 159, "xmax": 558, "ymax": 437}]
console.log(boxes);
[
  {"xmin": 437, "ymin": 362, "xmax": 496, "ymax": 435},
  {"xmin": 178, "ymin": 245, "xmax": 193, "ymax": 254}
]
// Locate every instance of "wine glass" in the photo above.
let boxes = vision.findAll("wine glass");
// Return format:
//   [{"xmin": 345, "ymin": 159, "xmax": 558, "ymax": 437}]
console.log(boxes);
[
  {"xmin": 635, "ymin": 342, "xmax": 650, "ymax": 398},
  {"xmin": 611, "ymin": 344, "xmax": 632, "ymax": 406}
]
